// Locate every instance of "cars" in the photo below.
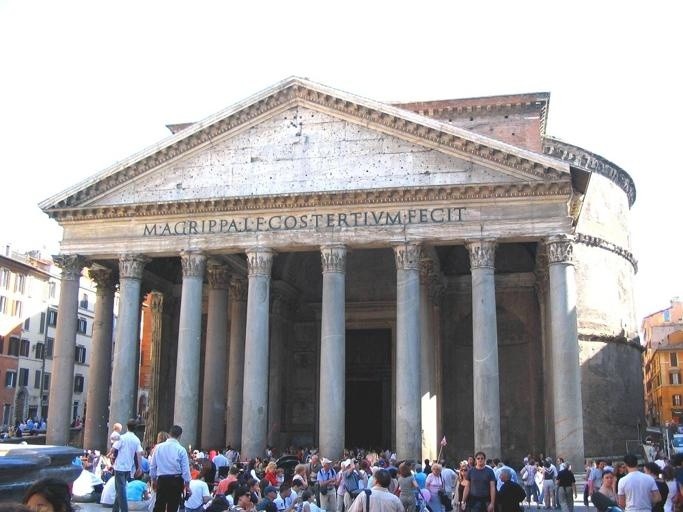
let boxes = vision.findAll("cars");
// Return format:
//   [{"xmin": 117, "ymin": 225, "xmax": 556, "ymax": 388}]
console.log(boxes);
[{"xmin": 670, "ymin": 434, "xmax": 682, "ymax": 459}]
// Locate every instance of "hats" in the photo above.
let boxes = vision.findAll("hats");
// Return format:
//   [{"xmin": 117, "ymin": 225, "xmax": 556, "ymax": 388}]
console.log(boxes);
[
  {"xmin": 321, "ymin": 458, "xmax": 332, "ymax": 466},
  {"xmin": 265, "ymin": 486, "xmax": 279, "ymax": 495}
]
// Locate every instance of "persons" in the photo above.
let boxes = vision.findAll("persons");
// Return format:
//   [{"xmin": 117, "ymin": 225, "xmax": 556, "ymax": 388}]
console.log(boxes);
[
  {"xmin": 497, "ymin": 469, "xmax": 526, "ymax": 512},
  {"xmin": 149, "ymin": 431, "xmax": 169, "ymax": 512},
  {"xmin": 20, "ymin": 476, "xmax": 74, "ymax": 512},
  {"xmin": 110, "ymin": 423, "xmax": 121, "ymax": 465},
  {"xmin": 150, "ymin": 425, "xmax": 192, "ymax": 512},
  {"xmin": 0, "ymin": 416, "xmax": 46, "ymax": 441},
  {"xmin": 127, "ymin": 449, "xmax": 152, "ymax": 512},
  {"xmin": 72, "ymin": 449, "xmax": 116, "ymax": 507},
  {"xmin": 639, "ymin": 444, "xmax": 683, "ymax": 512},
  {"xmin": 520, "ymin": 454, "xmax": 577, "ymax": 512},
  {"xmin": 462, "ymin": 452, "xmax": 496, "ymax": 512},
  {"xmin": 349, "ymin": 469, "xmax": 405, "ymax": 512},
  {"xmin": 184, "ymin": 446, "xmax": 475, "ymax": 512},
  {"xmin": 618, "ymin": 455, "xmax": 662, "ymax": 512},
  {"xmin": 111, "ymin": 421, "xmax": 143, "ymax": 512},
  {"xmin": 584, "ymin": 459, "xmax": 627, "ymax": 512},
  {"xmin": 486, "ymin": 458, "xmax": 517, "ymax": 492}
]
